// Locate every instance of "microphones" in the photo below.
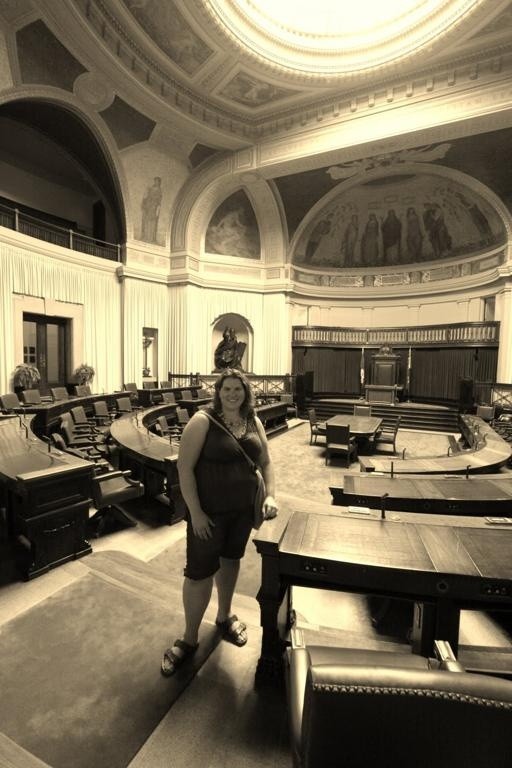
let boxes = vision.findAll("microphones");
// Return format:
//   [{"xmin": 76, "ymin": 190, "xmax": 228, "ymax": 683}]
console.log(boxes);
[
  {"xmin": 16, "ymin": 414, "xmax": 22, "ymax": 426},
  {"xmin": 464, "ymin": 410, "xmax": 488, "ymax": 449},
  {"xmin": 381, "ymin": 493, "xmax": 389, "ymax": 519},
  {"xmin": 447, "ymin": 446, "xmax": 452, "ymax": 455},
  {"xmin": 466, "ymin": 465, "xmax": 472, "ymax": 478},
  {"xmin": 402, "ymin": 448, "xmax": 407, "ymax": 459},
  {"xmin": 21, "ymin": 422, "xmax": 29, "ymax": 437},
  {"xmin": 133, "ymin": 398, "xmax": 172, "ymax": 445},
  {"xmin": 43, "ymin": 435, "xmax": 51, "ymax": 452}
]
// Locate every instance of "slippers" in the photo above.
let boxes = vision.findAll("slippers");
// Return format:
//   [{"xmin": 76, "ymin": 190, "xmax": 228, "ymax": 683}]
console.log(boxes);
[
  {"xmin": 161, "ymin": 635, "xmax": 199, "ymax": 677},
  {"xmin": 215, "ymin": 615, "xmax": 250, "ymax": 647}
]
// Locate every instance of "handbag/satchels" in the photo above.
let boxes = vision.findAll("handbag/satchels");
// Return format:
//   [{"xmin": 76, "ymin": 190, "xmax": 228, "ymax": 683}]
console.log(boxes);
[{"xmin": 253, "ymin": 468, "xmax": 269, "ymax": 532}]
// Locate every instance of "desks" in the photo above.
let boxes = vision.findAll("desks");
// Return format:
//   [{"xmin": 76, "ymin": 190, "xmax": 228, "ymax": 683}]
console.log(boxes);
[
  {"xmin": 249, "ymin": 504, "xmax": 512, "ymax": 696},
  {"xmin": 357, "ymin": 410, "xmax": 512, "ymax": 475},
  {"xmin": 338, "ymin": 469, "xmax": 511, "ymax": 665},
  {"xmin": 317, "ymin": 413, "xmax": 385, "ymax": 455}
]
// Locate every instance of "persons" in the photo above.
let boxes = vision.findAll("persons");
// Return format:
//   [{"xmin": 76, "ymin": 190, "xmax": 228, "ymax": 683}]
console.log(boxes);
[{"xmin": 161, "ymin": 367, "xmax": 273, "ymax": 676}]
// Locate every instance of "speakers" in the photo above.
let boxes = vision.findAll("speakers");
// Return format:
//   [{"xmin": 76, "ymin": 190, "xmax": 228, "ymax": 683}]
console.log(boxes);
[
  {"xmin": 459, "ymin": 376, "xmax": 473, "ymax": 407},
  {"xmin": 304, "ymin": 371, "xmax": 313, "ymax": 393}
]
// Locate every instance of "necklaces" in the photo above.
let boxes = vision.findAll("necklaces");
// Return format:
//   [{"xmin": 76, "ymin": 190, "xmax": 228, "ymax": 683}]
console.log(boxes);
[{"xmin": 217, "ymin": 408, "xmax": 253, "ymax": 433}]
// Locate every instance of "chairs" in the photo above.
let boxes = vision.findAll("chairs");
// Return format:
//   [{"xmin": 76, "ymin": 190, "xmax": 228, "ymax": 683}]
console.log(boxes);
[
  {"xmin": 352, "ymin": 404, "xmax": 371, "ymax": 419},
  {"xmin": 305, "ymin": 407, "xmax": 326, "ymax": 446},
  {"xmin": 0, "ymin": 379, "xmax": 297, "ymax": 594},
  {"xmin": 323, "ymin": 421, "xmax": 356, "ymax": 470},
  {"xmin": 367, "ymin": 414, "xmax": 403, "ymax": 455},
  {"xmin": 280, "ymin": 624, "xmax": 512, "ymax": 768}
]
